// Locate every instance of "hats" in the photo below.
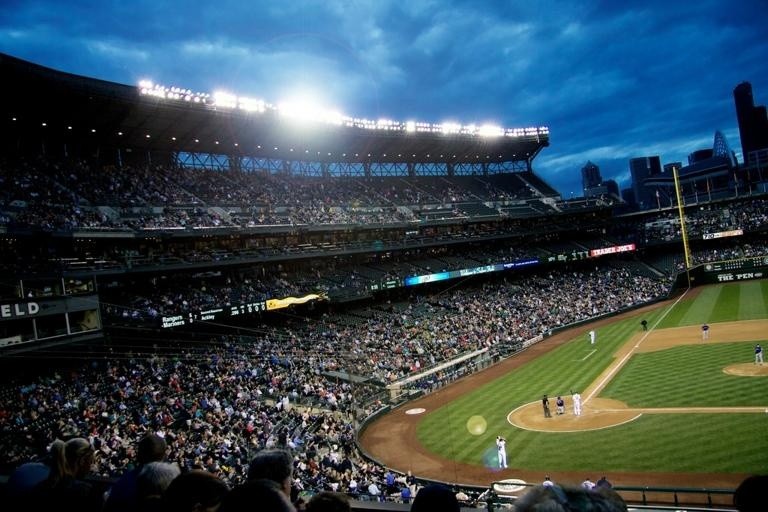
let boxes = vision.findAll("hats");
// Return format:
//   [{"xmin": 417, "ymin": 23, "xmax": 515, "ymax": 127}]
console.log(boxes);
[
  {"xmin": 8, "ymin": 461, "xmax": 55, "ymax": 498},
  {"xmin": 250, "ymin": 450, "xmax": 292, "ymax": 477}
]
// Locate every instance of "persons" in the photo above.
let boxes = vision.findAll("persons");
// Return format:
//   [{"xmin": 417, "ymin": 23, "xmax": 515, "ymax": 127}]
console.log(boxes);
[
  {"xmin": 555, "ymin": 396, "xmax": 564, "ymax": 415},
  {"xmin": 731, "ymin": 474, "xmax": 768, "ymax": 511},
  {"xmin": 495, "ymin": 436, "xmax": 508, "ymax": 468},
  {"xmin": 753, "ymin": 343, "xmax": 764, "ymax": 365},
  {"xmin": 359, "ymin": 455, "xmax": 629, "ymax": 511},
  {"xmin": 588, "ymin": 328, "xmax": 595, "ymax": 344},
  {"xmin": 558, "ymin": 193, "xmax": 767, "ymax": 329},
  {"xmin": 572, "ymin": 391, "xmax": 581, "ymax": 415},
  {"xmin": 701, "ymin": 323, "xmax": 709, "ymax": 340},
  {"xmin": 640, "ymin": 320, "xmax": 648, "ymax": 331}
]
